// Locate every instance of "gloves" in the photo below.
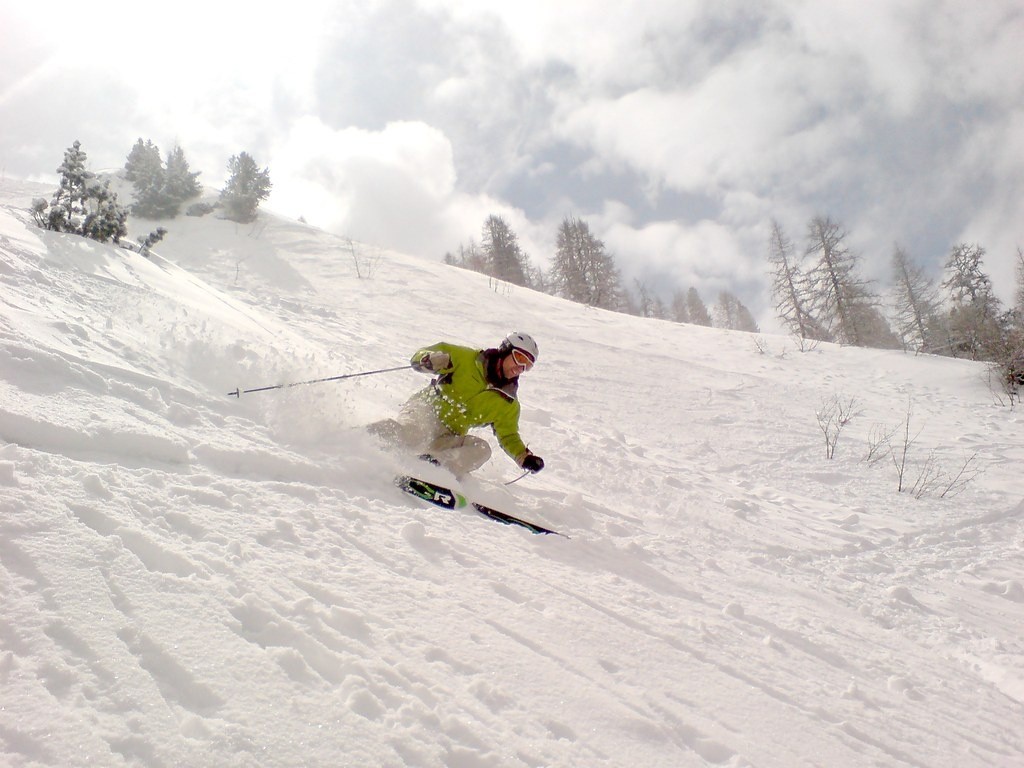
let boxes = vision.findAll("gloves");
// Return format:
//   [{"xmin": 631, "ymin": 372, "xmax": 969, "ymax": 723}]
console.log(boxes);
[
  {"xmin": 425, "ymin": 352, "xmax": 453, "ymax": 371},
  {"xmin": 522, "ymin": 455, "xmax": 544, "ymax": 475}
]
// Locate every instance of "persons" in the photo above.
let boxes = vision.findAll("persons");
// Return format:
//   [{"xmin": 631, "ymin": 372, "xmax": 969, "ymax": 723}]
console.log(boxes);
[{"xmin": 351, "ymin": 332, "xmax": 544, "ymax": 482}]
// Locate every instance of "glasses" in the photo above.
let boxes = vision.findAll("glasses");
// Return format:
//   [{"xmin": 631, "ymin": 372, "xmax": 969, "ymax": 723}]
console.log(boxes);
[{"xmin": 511, "ymin": 349, "xmax": 534, "ymax": 372}]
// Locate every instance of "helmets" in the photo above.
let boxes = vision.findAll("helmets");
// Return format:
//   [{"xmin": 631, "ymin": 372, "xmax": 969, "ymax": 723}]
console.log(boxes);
[{"xmin": 503, "ymin": 331, "xmax": 538, "ymax": 364}]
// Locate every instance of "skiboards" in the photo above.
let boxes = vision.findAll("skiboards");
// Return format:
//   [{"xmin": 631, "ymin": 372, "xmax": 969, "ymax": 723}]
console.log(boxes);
[{"xmin": 392, "ymin": 474, "xmax": 570, "ymax": 540}]
